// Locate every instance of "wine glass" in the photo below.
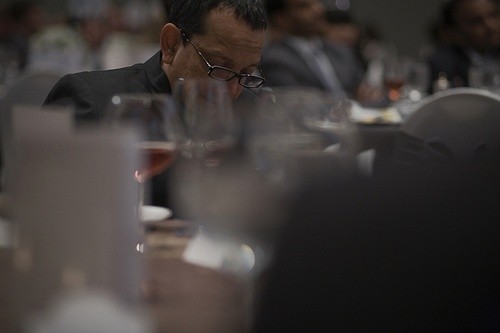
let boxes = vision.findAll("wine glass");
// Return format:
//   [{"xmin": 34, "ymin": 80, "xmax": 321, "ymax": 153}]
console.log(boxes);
[{"xmin": 105, "ymin": 91, "xmax": 185, "ymax": 254}]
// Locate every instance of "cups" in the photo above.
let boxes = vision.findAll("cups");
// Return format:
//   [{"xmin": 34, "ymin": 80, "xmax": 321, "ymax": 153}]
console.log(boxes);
[
  {"xmin": 384, "ymin": 64, "xmax": 405, "ymax": 102},
  {"xmin": 163, "ymin": 77, "xmax": 241, "ymax": 168}
]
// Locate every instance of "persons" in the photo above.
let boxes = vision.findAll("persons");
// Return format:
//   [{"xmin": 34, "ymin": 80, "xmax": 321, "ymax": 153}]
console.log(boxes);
[
  {"xmin": 261, "ymin": 0, "xmax": 500, "ymax": 122},
  {"xmin": 41, "ymin": 1, "xmax": 273, "ymax": 137}
]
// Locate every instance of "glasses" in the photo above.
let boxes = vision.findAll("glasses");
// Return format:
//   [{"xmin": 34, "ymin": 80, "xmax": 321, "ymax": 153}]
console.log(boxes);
[{"xmin": 179, "ymin": 28, "xmax": 267, "ymax": 90}]
